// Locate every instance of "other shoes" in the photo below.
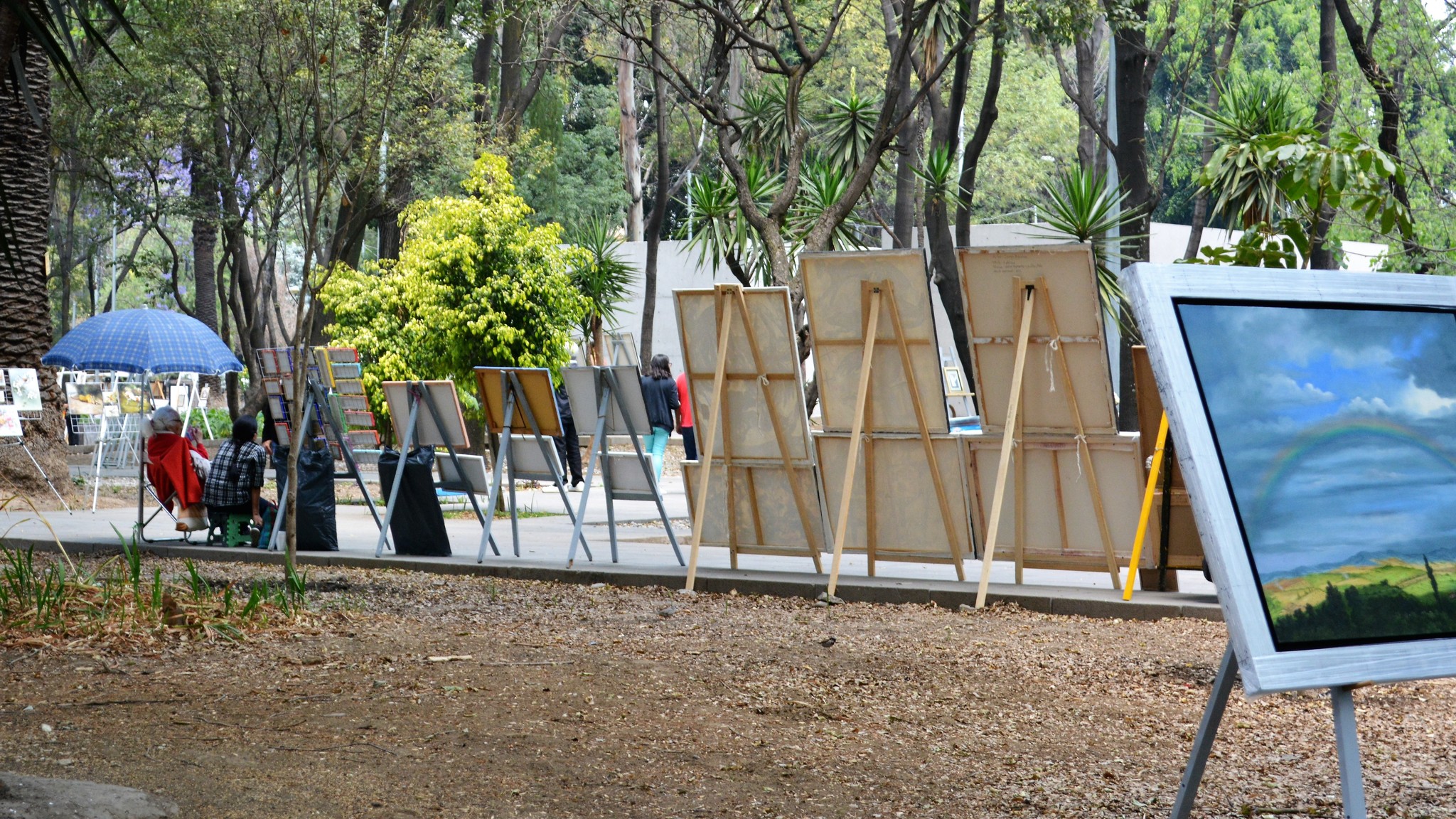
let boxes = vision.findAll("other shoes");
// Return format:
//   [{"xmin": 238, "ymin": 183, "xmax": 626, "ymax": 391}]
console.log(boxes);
[
  {"xmin": 568, "ymin": 481, "xmax": 584, "ymax": 492},
  {"xmin": 541, "ymin": 484, "xmax": 568, "ymax": 493},
  {"xmin": 250, "ymin": 525, "xmax": 261, "ymax": 548}
]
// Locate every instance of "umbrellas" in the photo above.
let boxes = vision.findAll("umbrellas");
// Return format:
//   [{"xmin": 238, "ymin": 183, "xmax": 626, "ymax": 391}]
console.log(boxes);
[{"xmin": 38, "ymin": 303, "xmax": 244, "ymax": 542}]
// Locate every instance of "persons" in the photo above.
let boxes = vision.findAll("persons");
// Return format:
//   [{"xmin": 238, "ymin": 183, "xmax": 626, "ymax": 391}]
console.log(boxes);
[
  {"xmin": 674, "ymin": 372, "xmax": 698, "ymax": 460},
  {"xmin": 588, "ymin": 341, "xmax": 607, "ymax": 367},
  {"xmin": 641, "ymin": 354, "xmax": 680, "ymax": 494},
  {"xmin": 140, "ymin": 404, "xmax": 212, "ymax": 514},
  {"xmin": 542, "ymin": 364, "xmax": 582, "ymax": 493},
  {"xmin": 202, "ymin": 414, "xmax": 278, "ymax": 551},
  {"xmin": 261, "ymin": 347, "xmax": 318, "ymax": 531}
]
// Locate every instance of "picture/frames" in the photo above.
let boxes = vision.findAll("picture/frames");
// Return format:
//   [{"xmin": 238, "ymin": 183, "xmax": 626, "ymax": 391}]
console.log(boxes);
[{"xmin": 1116, "ymin": 258, "xmax": 1456, "ymax": 697}]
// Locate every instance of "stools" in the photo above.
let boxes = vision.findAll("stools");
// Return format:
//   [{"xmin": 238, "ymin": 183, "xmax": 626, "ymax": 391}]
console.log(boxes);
[{"xmin": 205, "ymin": 513, "xmax": 273, "ymax": 550}]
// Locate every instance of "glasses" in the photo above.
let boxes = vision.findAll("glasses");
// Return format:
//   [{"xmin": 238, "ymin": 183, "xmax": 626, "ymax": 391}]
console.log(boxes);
[{"xmin": 668, "ymin": 363, "xmax": 672, "ymax": 366}]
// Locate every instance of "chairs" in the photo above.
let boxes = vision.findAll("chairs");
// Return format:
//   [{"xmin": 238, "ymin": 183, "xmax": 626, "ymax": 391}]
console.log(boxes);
[{"xmin": 143, "ymin": 461, "xmax": 205, "ymax": 547}]
